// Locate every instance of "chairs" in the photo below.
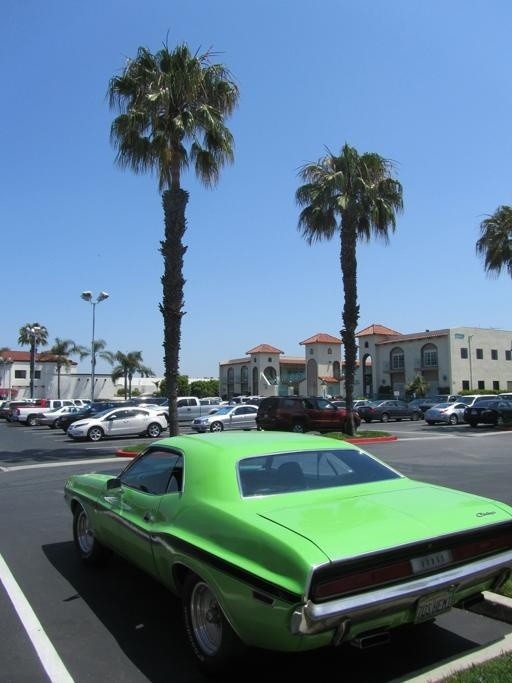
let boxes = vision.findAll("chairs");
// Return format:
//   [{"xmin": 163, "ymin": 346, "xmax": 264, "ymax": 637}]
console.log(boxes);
[{"xmin": 277, "ymin": 460, "xmax": 305, "ymax": 490}]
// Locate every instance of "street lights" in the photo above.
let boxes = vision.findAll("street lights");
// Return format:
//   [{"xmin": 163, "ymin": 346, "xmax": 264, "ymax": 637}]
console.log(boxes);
[
  {"xmin": 25, "ymin": 321, "xmax": 46, "ymax": 399},
  {"xmin": 466, "ymin": 333, "xmax": 474, "ymax": 392},
  {"xmin": 78, "ymin": 289, "xmax": 109, "ymax": 409},
  {"xmin": 320, "ymin": 379, "xmax": 329, "ymax": 398}
]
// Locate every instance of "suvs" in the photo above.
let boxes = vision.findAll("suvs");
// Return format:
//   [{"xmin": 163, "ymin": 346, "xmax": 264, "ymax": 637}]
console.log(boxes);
[
  {"xmin": 254, "ymin": 395, "xmax": 361, "ymax": 433},
  {"xmin": 432, "ymin": 393, "xmax": 512, "ymax": 409}
]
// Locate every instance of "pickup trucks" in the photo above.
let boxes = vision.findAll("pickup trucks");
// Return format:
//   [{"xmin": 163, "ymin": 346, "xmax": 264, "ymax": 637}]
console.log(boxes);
[
  {"xmin": 1, "ymin": 398, "xmax": 93, "ymax": 426},
  {"xmin": 160, "ymin": 396, "xmax": 223, "ymax": 421}
]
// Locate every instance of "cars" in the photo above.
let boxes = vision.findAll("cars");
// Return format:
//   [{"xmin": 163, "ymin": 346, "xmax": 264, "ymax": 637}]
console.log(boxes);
[
  {"xmin": 191, "ymin": 404, "xmax": 259, "ymax": 433},
  {"xmin": 358, "ymin": 399, "xmax": 422, "ymax": 423},
  {"xmin": 67, "ymin": 406, "xmax": 166, "ymax": 438},
  {"xmin": 64, "ymin": 427, "xmax": 512, "ymax": 669},
  {"xmin": 423, "ymin": 402, "xmax": 469, "ymax": 425},
  {"xmin": 218, "ymin": 394, "xmax": 267, "ymax": 406},
  {"xmin": 463, "ymin": 399, "xmax": 512, "ymax": 426},
  {"xmin": 38, "ymin": 405, "xmax": 102, "ymax": 429},
  {"xmin": 326, "ymin": 398, "xmax": 371, "ymax": 412},
  {"xmin": 409, "ymin": 398, "xmax": 441, "ymax": 412}
]
